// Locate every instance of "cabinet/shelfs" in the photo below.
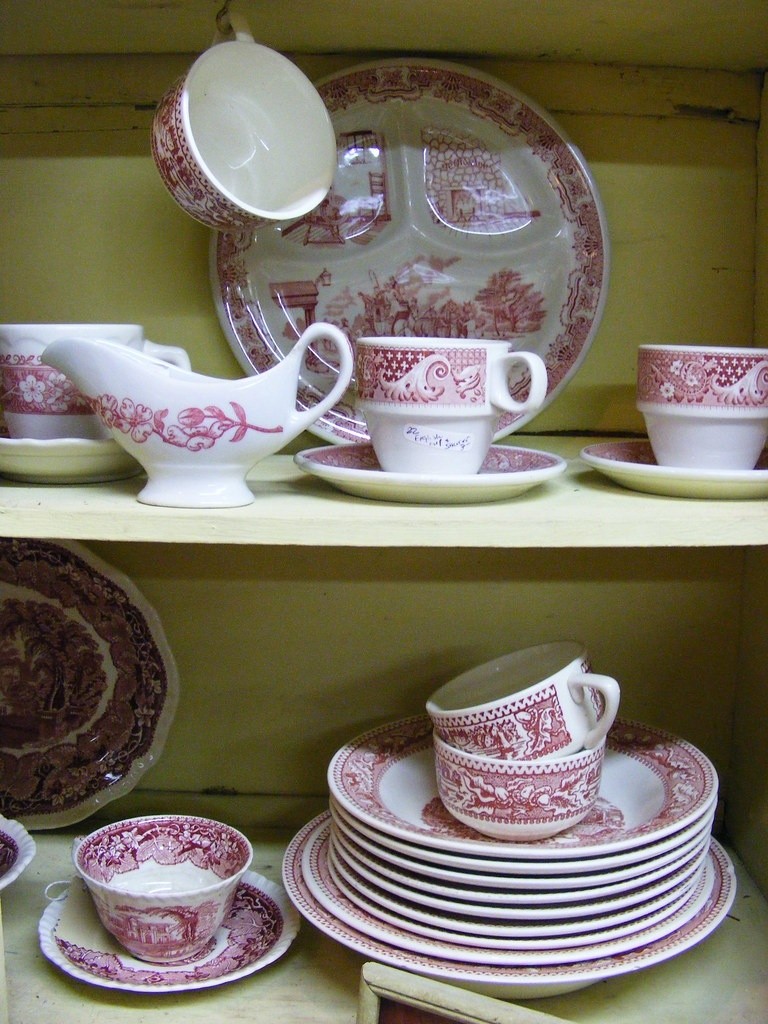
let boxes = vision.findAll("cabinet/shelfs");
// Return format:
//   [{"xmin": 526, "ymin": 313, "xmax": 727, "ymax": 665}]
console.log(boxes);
[{"xmin": 0, "ymin": 44, "xmax": 768, "ymax": 1024}]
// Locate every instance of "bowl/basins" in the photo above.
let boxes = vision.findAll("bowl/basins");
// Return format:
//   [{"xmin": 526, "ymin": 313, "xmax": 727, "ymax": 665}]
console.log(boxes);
[{"xmin": 0, "ymin": 813, "xmax": 37, "ymax": 895}]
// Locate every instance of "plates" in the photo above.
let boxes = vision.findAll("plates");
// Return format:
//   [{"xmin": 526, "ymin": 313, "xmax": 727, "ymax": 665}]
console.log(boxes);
[
  {"xmin": 0, "ymin": 436, "xmax": 142, "ymax": 484},
  {"xmin": 209, "ymin": 58, "xmax": 610, "ymax": 442},
  {"xmin": 579, "ymin": 442, "xmax": 768, "ymax": 501},
  {"xmin": 0, "ymin": 536, "xmax": 179, "ymax": 829},
  {"xmin": 38, "ymin": 870, "xmax": 301, "ymax": 993},
  {"xmin": 280, "ymin": 715, "xmax": 737, "ymax": 998},
  {"xmin": 293, "ymin": 442, "xmax": 567, "ymax": 502}
]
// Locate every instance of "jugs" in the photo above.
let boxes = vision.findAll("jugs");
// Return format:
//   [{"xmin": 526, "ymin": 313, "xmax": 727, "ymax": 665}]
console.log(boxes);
[{"xmin": 43, "ymin": 322, "xmax": 354, "ymax": 508}]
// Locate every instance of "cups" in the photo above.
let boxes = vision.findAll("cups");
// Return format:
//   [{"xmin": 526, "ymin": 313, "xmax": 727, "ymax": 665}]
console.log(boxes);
[
  {"xmin": 355, "ymin": 335, "xmax": 548, "ymax": 473},
  {"xmin": 0, "ymin": 322, "xmax": 191, "ymax": 441},
  {"xmin": 74, "ymin": 814, "xmax": 254, "ymax": 962},
  {"xmin": 150, "ymin": 7, "xmax": 337, "ymax": 232},
  {"xmin": 426, "ymin": 640, "xmax": 621, "ymax": 759},
  {"xmin": 635, "ymin": 343, "xmax": 768, "ymax": 470},
  {"xmin": 433, "ymin": 728, "xmax": 607, "ymax": 841}
]
